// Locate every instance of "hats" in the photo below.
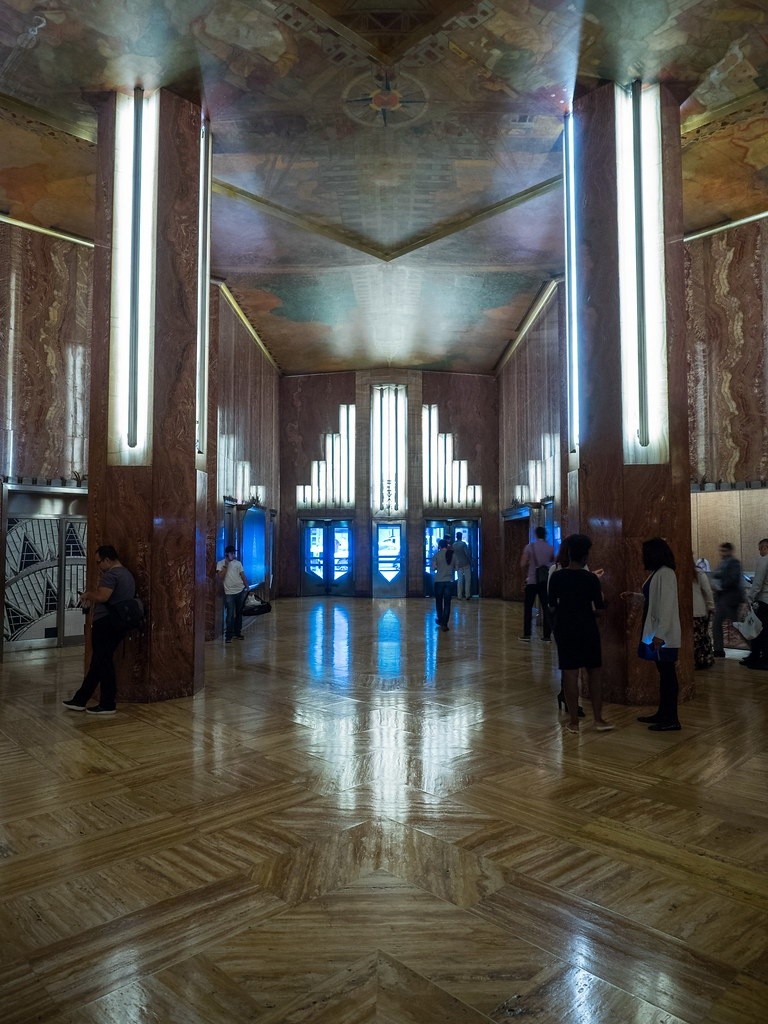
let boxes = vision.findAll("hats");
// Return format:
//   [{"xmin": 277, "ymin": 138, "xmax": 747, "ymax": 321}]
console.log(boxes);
[{"xmin": 224, "ymin": 544, "xmax": 238, "ymax": 554}]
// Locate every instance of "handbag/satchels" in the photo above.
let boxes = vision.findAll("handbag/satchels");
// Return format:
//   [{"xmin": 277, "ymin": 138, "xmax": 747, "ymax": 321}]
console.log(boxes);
[
  {"xmin": 241, "ymin": 591, "xmax": 272, "ymax": 616},
  {"xmin": 732, "ymin": 605, "xmax": 763, "ymax": 640},
  {"xmin": 111, "ymin": 598, "xmax": 144, "ymax": 630},
  {"xmin": 536, "ymin": 565, "xmax": 549, "ymax": 589}
]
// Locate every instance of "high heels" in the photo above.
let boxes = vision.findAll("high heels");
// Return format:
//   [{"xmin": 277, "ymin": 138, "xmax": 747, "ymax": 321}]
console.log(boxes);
[
  {"xmin": 557, "ymin": 691, "xmax": 583, "ymax": 710},
  {"xmin": 564, "ymin": 697, "xmax": 585, "ymax": 717}
]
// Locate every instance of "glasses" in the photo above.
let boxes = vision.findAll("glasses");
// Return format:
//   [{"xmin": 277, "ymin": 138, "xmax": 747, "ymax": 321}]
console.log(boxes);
[{"xmin": 96, "ymin": 557, "xmax": 105, "ymax": 565}]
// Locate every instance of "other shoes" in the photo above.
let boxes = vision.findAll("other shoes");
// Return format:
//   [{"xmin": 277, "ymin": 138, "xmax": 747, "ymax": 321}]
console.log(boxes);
[
  {"xmin": 648, "ymin": 721, "xmax": 681, "ymax": 732},
  {"xmin": 467, "ymin": 596, "xmax": 470, "ymax": 600},
  {"xmin": 225, "ymin": 638, "xmax": 231, "ymax": 643},
  {"xmin": 441, "ymin": 625, "xmax": 449, "ymax": 632},
  {"xmin": 637, "ymin": 714, "xmax": 663, "ymax": 723},
  {"xmin": 738, "ymin": 655, "xmax": 768, "ymax": 671},
  {"xmin": 457, "ymin": 597, "xmax": 461, "ymax": 600},
  {"xmin": 596, "ymin": 722, "xmax": 616, "ymax": 730},
  {"xmin": 540, "ymin": 638, "xmax": 552, "ymax": 643},
  {"xmin": 713, "ymin": 650, "xmax": 726, "ymax": 658},
  {"xmin": 565, "ymin": 722, "xmax": 578, "ymax": 734},
  {"xmin": 435, "ymin": 619, "xmax": 444, "ymax": 626},
  {"xmin": 518, "ymin": 636, "xmax": 530, "ymax": 642},
  {"xmin": 235, "ymin": 633, "xmax": 245, "ymax": 640}
]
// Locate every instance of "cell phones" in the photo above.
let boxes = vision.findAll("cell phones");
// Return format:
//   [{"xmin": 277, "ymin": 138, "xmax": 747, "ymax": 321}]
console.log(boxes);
[
  {"xmin": 77, "ymin": 590, "xmax": 84, "ymax": 596},
  {"xmin": 744, "ymin": 574, "xmax": 751, "ymax": 582}
]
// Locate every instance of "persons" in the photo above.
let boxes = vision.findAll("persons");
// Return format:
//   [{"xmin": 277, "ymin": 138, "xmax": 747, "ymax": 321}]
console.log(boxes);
[
  {"xmin": 519, "ymin": 527, "xmax": 554, "ymax": 642},
  {"xmin": 691, "ymin": 539, "xmax": 768, "ymax": 672},
  {"xmin": 215, "ymin": 546, "xmax": 251, "ymax": 643},
  {"xmin": 619, "ymin": 537, "xmax": 682, "ymax": 732},
  {"xmin": 432, "ymin": 531, "xmax": 472, "ymax": 632},
  {"xmin": 62, "ymin": 545, "xmax": 136, "ymax": 715},
  {"xmin": 547, "ymin": 534, "xmax": 617, "ymax": 734}
]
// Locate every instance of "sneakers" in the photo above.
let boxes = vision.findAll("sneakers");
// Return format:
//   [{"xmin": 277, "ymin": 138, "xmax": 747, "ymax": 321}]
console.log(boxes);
[
  {"xmin": 86, "ymin": 700, "xmax": 116, "ymax": 715},
  {"xmin": 62, "ymin": 697, "xmax": 86, "ymax": 710}
]
